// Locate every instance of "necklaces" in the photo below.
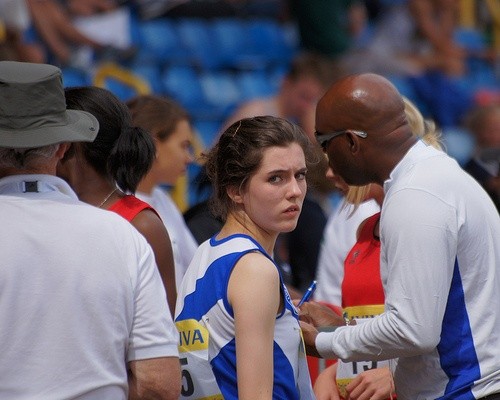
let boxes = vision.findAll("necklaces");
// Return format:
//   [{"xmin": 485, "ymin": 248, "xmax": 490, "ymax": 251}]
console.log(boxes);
[{"xmin": 96, "ymin": 188, "xmax": 119, "ymax": 208}]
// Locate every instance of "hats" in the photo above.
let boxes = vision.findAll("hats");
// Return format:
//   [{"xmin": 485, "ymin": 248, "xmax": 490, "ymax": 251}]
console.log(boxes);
[{"xmin": 0, "ymin": 61, "xmax": 99, "ymax": 149}]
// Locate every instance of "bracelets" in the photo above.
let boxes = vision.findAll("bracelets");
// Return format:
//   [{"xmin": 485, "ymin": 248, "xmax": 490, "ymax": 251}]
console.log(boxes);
[{"xmin": 344, "ymin": 318, "xmax": 351, "ymax": 327}]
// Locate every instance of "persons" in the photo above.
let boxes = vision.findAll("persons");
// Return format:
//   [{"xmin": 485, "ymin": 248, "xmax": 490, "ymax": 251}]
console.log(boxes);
[
  {"xmin": 0, "ymin": 60, "xmax": 182, "ymax": 399},
  {"xmin": 213, "ymin": 0, "xmax": 500, "ymax": 212},
  {"xmin": 312, "ymin": 96, "xmax": 449, "ymax": 399},
  {"xmin": 291, "ymin": 72, "xmax": 500, "ymax": 400},
  {"xmin": 126, "ymin": 94, "xmax": 198, "ymax": 293},
  {"xmin": 173, "ymin": 116, "xmax": 329, "ymax": 398},
  {"xmin": 57, "ymin": 85, "xmax": 177, "ymax": 321},
  {"xmin": 0, "ymin": 0, "xmax": 165, "ymax": 92}
]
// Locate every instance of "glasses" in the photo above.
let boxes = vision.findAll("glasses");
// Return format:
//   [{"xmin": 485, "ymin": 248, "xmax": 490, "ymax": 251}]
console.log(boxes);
[{"xmin": 314, "ymin": 130, "xmax": 367, "ymax": 153}]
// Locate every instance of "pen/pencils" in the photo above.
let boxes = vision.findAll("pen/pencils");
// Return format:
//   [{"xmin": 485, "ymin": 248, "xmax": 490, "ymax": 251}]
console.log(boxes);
[{"xmin": 297, "ymin": 280, "xmax": 318, "ymax": 309}]
